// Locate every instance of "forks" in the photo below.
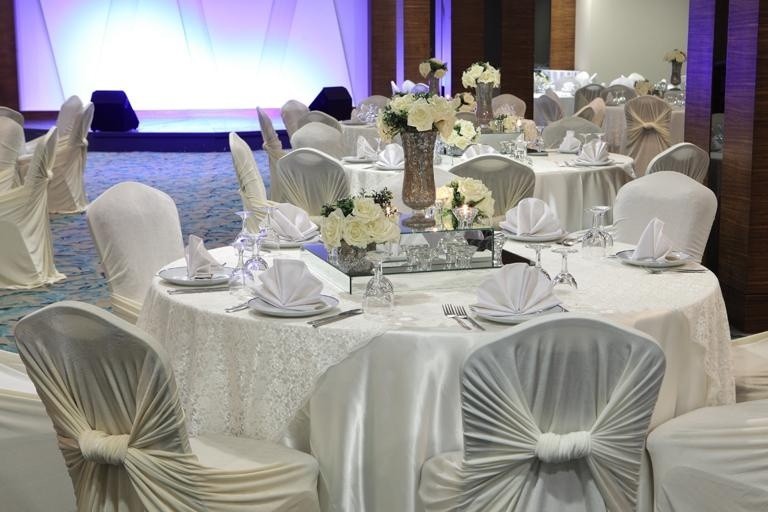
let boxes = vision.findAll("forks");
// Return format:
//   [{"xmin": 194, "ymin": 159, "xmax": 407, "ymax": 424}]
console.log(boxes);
[
  {"xmin": 440, "ymin": 302, "xmax": 471, "ymax": 331},
  {"xmin": 455, "ymin": 306, "xmax": 486, "ymax": 331}
]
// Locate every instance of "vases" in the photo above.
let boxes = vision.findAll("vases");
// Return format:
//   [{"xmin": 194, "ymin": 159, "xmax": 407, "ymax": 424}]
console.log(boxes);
[{"xmin": 669, "ymin": 59, "xmax": 682, "ymax": 86}]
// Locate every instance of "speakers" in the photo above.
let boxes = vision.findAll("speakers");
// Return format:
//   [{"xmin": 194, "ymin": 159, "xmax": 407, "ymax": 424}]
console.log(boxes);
[
  {"xmin": 308, "ymin": 87, "xmax": 352, "ymax": 121},
  {"xmin": 91, "ymin": 91, "xmax": 140, "ymax": 132}
]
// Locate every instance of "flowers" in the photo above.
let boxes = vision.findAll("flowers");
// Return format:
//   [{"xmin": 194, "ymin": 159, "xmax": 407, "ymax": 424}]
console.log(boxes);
[
  {"xmin": 460, "ymin": 61, "xmax": 502, "ymax": 90},
  {"xmin": 662, "ymin": 48, "xmax": 687, "ymax": 64},
  {"xmin": 417, "ymin": 56, "xmax": 449, "ymax": 79}
]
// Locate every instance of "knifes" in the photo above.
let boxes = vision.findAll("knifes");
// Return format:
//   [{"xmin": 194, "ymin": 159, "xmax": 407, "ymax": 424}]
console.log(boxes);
[
  {"xmin": 642, "ymin": 262, "xmax": 712, "ymax": 274},
  {"xmin": 309, "ymin": 308, "xmax": 363, "ymax": 328},
  {"xmin": 166, "ymin": 288, "xmax": 232, "ymax": 295}
]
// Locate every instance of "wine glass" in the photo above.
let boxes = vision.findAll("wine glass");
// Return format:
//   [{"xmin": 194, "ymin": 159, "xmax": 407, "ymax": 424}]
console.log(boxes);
[
  {"xmin": 552, "ymin": 246, "xmax": 580, "ymax": 306},
  {"xmin": 524, "ymin": 241, "xmax": 550, "ymax": 280},
  {"xmin": 598, "ymin": 79, "xmax": 686, "ymax": 110},
  {"xmin": 245, "ymin": 228, "xmax": 270, "ymax": 289},
  {"xmin": 227, "ymin": 234, "xmax": 256, "ymax": 296},
  {"xmin": 361, "ymin": 248, "xmax": 395, "ymax": 319},
  {"xmin": 579, "ymin": 203, "xmax": 613, "ymax": 262}
]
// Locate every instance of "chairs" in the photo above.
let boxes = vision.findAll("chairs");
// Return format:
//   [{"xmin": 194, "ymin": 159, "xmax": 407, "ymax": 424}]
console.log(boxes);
[
  {"xmin": 229, "ymin": 93, "xmax": 709, "ymax": 222},
  {"xmin": 534, "ymin": 71, "xmax": 685, "ymax": 176},
  {"xmin": 1, "ymin": 171, "xmax": 768, "ymax": 510},
  {"xmin": 1, "ymin": 95, "xmax": 94, "ymax": 291}
]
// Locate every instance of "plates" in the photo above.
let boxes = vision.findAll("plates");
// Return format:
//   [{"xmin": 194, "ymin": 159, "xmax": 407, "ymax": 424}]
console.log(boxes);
[
  {"xmin": 471, "ymin": 299, "xmax": 567, "ymax": 324},
  {"xmin": 340, "ymin": 129, "xmax": 620, "ymax": 173},
  {"xmin": 499, "ymin": 228, "xmax": 563, "ymax": 242},
  {"xmin": 161, "ymin": 263, "xmax": 232, "ymax": 285},
  {"xmin": 249, "ymin": 232, "xmax": 320, "ymax": 248},
  {"xmin": 249, "ymin": 292, "xmax": 336, "ymax": 317},
  {"xmin": 609, "ymin": 246, "xmax": 690, "ymax": 265}
]
